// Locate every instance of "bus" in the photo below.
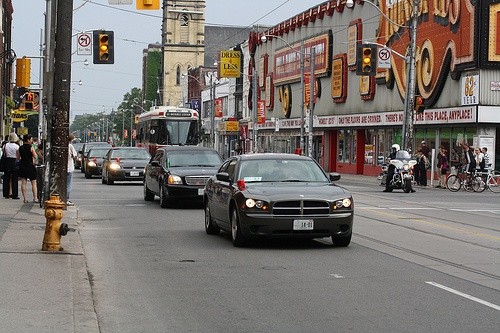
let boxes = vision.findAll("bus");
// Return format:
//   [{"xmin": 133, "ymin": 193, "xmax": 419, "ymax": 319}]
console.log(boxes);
[{"xmin": 135, "ymin": 106, "xmax": 206, "ymax": 150}]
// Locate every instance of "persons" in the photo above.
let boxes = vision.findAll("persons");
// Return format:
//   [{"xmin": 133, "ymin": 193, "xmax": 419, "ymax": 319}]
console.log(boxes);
[
  {"xmin": 382, "ymin": 141, "xmax": 489, "ymax": 193},
  {"xmin": 0, "ymin": 131, "xmax": 77, "ymax": 206}
]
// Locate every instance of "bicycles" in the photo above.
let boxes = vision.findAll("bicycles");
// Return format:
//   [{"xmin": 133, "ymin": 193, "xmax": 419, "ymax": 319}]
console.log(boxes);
[{"xmin": 446, "ymin": 162, "xmax": 500, "ymax": 193}]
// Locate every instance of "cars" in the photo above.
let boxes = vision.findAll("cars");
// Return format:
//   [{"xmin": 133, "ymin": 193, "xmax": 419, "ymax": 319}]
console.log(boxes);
[
  {"xmin": 102, "ymin": 146, "xmax": 153, "ymax": 186},
  {"xmin": 143, "ymin": 145, "xmax": 225, "ymax": 208},
  {"xmin": 84, "ymin": 147, "xmax": 111, "ymax": 179},
  {"xmin": 71, "ymin": 141, "xmax": 112, "ymax": 173},
  {"xmin": 365, "ymin": 151, "xmax": 385, "ymax": 163},
  {"xmin": 203, "ymin": 152, "xmax": 354, "ymax": 248}
]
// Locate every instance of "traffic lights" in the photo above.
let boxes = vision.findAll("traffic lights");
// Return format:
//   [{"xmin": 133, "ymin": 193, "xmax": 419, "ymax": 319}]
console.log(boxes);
[
  {"xmin": 93, "ymin": 29, "xmax": 115, "ymax": 64},
  {"xmin": 355, "ymin": 43, "xmax": 377, "ymax": 76},
  {"xmin": 24, "ymin": 91, "xmax": 35, "ymax": 110},
  {"xmin": 416, "ymin": 96, "xmax": 426, "ymax": 113}
]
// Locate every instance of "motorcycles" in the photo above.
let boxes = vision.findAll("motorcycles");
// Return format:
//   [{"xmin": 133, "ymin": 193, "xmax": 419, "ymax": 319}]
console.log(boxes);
[{"xmin": 389, "ymin": 149, "xmax": 418, "ymax": 193}]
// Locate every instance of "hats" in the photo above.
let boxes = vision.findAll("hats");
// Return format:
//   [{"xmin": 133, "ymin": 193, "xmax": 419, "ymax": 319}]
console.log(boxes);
[{"xmin": 8, "ymin": 132, "xmax": 19, "ymax": 141}]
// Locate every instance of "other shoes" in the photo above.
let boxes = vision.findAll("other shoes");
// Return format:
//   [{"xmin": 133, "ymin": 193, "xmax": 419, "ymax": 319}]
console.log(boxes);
[
  {"xmin": 6, "ymin": 195, "xmax": 10, "ymax": 199},
  {"xmin": 411, "ymin": 189, "xmax": 416, "ymax": 193},
  {"xmin": 66, "ymin": 201, "xmax": 74, "ymax": 206},
  {"xmin": 24, "ymin": 200, "xmax": 28, "ymax": 203},
  {"xmin": 383, "ymin": 189, "xmax": 389, "ymax": 192},
  {"xmin": 33, "ymin": 199, "xmax": 39, "ymax": 203},
  {"xmin": 436, "ymin": 185, "xmax": 441, "ymax": 188},
  {"xmin": 14, "ymin": 197, "xmax": 20, "ymax": 199},
  {"xmin": 441, "ymin": 186, "xmax": 446, "ymax": 189}
]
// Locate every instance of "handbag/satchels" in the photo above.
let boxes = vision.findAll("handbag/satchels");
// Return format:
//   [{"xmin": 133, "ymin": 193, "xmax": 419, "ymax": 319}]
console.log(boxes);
[{"xmin": 0, "ymin": 143, "xmax": 7, "ymax": 172}]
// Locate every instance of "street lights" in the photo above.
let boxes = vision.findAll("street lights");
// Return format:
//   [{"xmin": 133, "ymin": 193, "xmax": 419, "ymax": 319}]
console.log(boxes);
[
  {"xmin": 212, "ymin": 60, "xmax": 259, "ymax": 151},
  {"xmin": 86, "ymin": 117, "xmax": 109, "ymax": 142},
  {"xmin": 261, "ymin": 32, "xmax": 316, "ymax": 159},
  {"xmin": 347, "ymin": 0, "xmax": 419, "ymax": 153},
  {"xmin": 181, "ymin": 73, "xmax": 213, "ymax": 148}
]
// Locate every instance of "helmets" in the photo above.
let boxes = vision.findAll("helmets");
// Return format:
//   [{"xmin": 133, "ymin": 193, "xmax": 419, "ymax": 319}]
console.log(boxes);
[{"xmin": 392, "ymin": 144, "xmax": 400, "ymax": 151}]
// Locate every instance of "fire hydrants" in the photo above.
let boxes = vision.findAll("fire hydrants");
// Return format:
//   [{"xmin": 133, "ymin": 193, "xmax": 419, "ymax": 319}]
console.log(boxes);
[{"xmin": 41, "ymin": 193, "xmax": 69, "ymax": 252}]
